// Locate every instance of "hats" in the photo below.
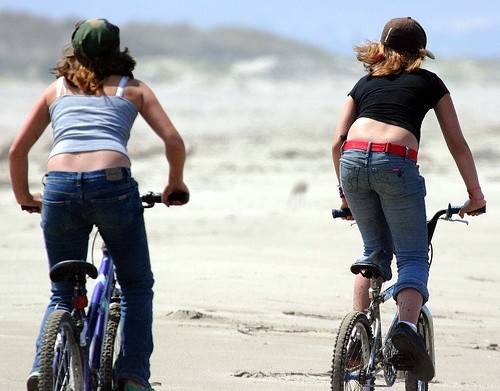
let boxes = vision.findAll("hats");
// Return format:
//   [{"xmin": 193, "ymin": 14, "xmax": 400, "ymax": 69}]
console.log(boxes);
[
  {"xmin": 71, "ymin": 17, "xmax": 120, "ymax": 61},
  {"xmin": 381, "ymin": 17, "xmax": 435, "ymax": 60}
]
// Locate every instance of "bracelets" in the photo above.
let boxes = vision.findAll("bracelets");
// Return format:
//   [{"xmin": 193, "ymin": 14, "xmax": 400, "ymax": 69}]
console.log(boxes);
[
  {"xmin": 339, "ymin": 187, "xmax": 344, "ymax": 198},
  {"xmin": 468, "ymin": 187, "xmax": 484, "ymax": 200}
]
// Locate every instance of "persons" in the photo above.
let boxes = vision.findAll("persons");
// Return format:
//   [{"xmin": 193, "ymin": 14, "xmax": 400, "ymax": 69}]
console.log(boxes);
[
  {"xmin": 332, "ymin": 16, "xmax": 486, "ymax": 380},
  {"xmin": 9, "ymin": 17, "xmax": 190, "ymax": 391}
]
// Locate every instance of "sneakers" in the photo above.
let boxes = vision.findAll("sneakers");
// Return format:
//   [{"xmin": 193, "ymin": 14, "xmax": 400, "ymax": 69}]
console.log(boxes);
[
  {"xmin": 346, "ymin": 339, "xmax": 364, "ymax": 366},
  {"xmin": 391, "ymin": 322, "xmax": 435, "ymax": 382}
]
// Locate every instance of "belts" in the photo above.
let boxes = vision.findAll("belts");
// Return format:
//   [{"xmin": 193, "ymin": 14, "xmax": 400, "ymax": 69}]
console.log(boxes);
[{"xmin": 343, "ymin": 140, "xmax": 417, "ymax": 163}]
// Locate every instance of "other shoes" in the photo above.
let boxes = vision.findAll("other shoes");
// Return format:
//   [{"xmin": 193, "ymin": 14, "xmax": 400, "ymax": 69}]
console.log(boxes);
[
  {"xmin": 121, "ymin": 378, "xmax": 155, "ymax": 391},
  {"xmin": 26, "ymin": 370, "xmax": 41, "ymax": 391}
]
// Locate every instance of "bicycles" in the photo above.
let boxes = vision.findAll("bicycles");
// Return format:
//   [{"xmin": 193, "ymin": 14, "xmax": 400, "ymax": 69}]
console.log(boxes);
[
  {"xmin": 21, "ymin": 191, "xmax": 189, "ymax": 391},
  {"xmin": 330, "ymin": 204, "xmax": 486, "ymax": 391}
]
